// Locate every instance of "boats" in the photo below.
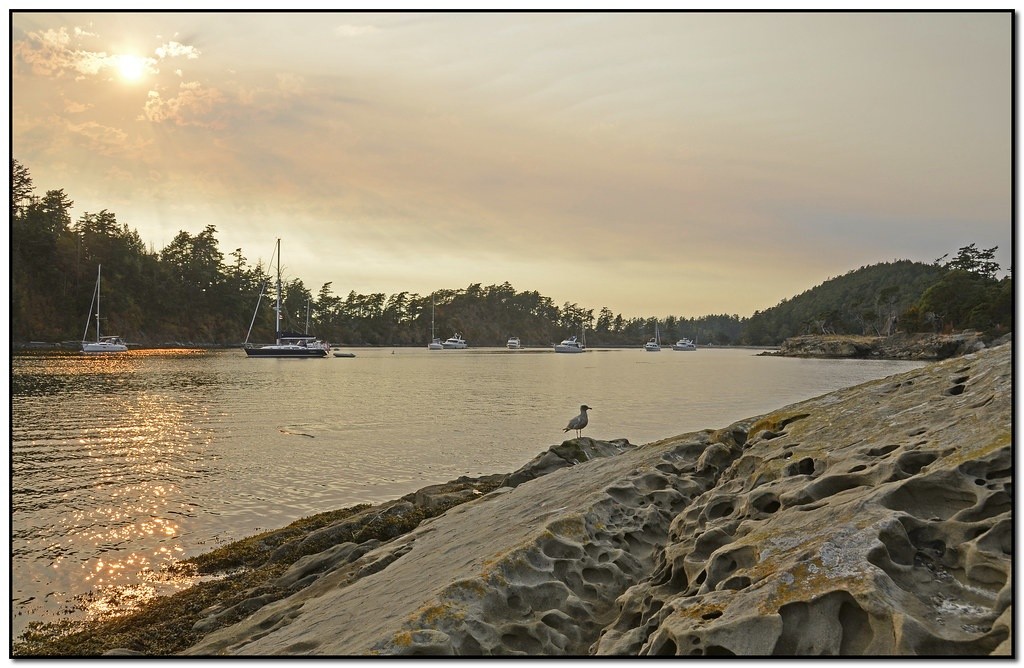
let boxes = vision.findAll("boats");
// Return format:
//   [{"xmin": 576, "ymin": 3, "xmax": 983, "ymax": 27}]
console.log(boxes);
[
  {"xmin": 645, "ymin": 337, "xmax": 661, "ymax": 352},
  {"xmin": 672, "ymin": 338, "xmax": 696, "ymax": 350},
  {"xmin": 425, "ymin": 296, "xmax": 443, "ymax": 349},
  {"xmin": 240, "ymin": 238, "xmax": 330, "ymax": 358},
  {"xmin": 333, "ymin": 352, "xmax": 356, "ymax": 358},
  {"xmin": 507, "ymin": 335, "xmax": 521, "ymax": 349},
  {"xmin": 442, "ymin": 332, "xmax": 468, "ymax": 349},
  {"xmin": 553, "ymin": 336, "xmax": 582, "ymax": 353},
  {"xmin": 81, "ymin": 264, "xmax": 129, "ymax": 352}
]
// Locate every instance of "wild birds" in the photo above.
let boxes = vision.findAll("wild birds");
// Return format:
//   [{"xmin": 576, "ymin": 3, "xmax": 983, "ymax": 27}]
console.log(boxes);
[{"xmin": 562, "ymin": 405, "xmax": 593, "ymax": 438}]
